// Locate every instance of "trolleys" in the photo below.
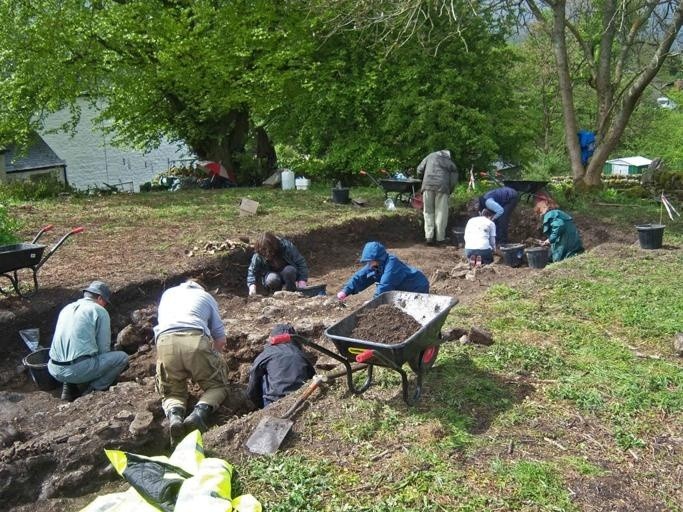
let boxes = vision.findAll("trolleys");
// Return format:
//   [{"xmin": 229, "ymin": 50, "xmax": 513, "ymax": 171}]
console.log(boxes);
[
  {"xmin": 0, "ymin": 224, "xmax": 84, "ymax": 299},
  {"xmin": 269, "ymin": 290, "xmax": 460, "ymax": 405},
  {"xmin": 478, "ymin": 174, "xmax": 551, "ymax": 205},
  {"xmin": 359, "ymin": 169, "xmax": 423, "ymax": 207}
]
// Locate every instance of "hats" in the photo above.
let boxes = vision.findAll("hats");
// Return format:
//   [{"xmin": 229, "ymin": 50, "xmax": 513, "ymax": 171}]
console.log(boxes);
[{"xmin": 82, "ymin": 280, "xmax": 113, "ymax": 306}]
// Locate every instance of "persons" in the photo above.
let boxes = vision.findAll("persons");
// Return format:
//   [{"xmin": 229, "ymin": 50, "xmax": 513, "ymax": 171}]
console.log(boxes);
[
  {"xmin": 534, "ymin": 200, "xmax": 585, "ymax": 262},
  {"xmin": 465, "ymin": 210, "xmax": 497, "ymax": 269},
  {"xmin": 151, "ymin": 274, "xmax": 227, "ymax": 450},
  {"xmin": 416, "ymin": 149, "xmax": 459, "ymax": 246},
  {"xmin": 338, "ymin": 242, "xmax": 430, "ymax": 305},
  {"xmin": 466, "ymin": 188, "xmax": 521, "ymax": 243},
  {"xmin": 246, "ymin": 232, "xmax": 309, "ymax": 298},
  {"xmin": 47, "ymin": 281, "xmax": 130, "ymax": 401},
  {"xmin": 246, "ymin": 326, "xmax": 317, "ymax": 408}
]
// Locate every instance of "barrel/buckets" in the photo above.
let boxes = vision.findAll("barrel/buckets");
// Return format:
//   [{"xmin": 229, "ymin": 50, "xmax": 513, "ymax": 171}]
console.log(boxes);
[
  {"xmin": 21, "ymin": 347, "xmax": 62, "ymax": 390},
  {"xmin": 332, "ymin": 187, "xmax": 350, "ymax": 205},
  {"xmin": 499, "ymin": 243, "xmax": 525, "ymax": 267},
  {"xmin": 634, "ymin": 224, "xmax": 666, "ymax": 249},
  {"xmin": 282, "ymin": 168, "xmax": 294, "ymax": 191},
  {"xmin": 449, "ymin": 227, "xmax": 465, "ymax": 246},
  {"xmin": 524, "ymin": 246, "xmax": 549, "ymax": 269},
  {"xmin": 297, "ymin": 283, "xmax": 327, "ymax": 297}
]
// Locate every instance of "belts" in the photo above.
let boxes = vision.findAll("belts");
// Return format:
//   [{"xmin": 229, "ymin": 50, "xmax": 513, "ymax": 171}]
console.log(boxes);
[{"xmin": 52, "ymin": 355, "xmax": 91, "ymax": 365}]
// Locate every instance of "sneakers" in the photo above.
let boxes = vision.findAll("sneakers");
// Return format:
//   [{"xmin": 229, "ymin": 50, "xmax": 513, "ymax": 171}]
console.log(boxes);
[
  {"xmin": 61, "ymin": 384, "xmax": 82, "ymax": 401},
  {"xmin": 438, "ymin": 239, "xmax": 448, "ymax": 246},
  {"xmin": 470, "ymin": 255, "xmax": 476, "ymax": 269},
  {"xmin": 183, "ymin": 412, "xmax": 209, "ymax": 435},
  {"xmin": 87, "ymin": 379, "xmax": 117, "ymax": 394},
  {"xmin": 170, "ymin": 415, "xmax": 185, "ymax": 454},
  {"xmin": 427, "ymin": 238, "xmax": 434, "ymax": 246},
  {"xmin": 474, "ymin": 256, "xmax": 482, "ymax": 268}
]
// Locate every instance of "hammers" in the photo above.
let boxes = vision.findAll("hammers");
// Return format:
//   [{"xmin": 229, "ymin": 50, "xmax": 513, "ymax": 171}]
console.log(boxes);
[{"xmin": 322, "ymin": 364, "xmax": 367, "ymax": 383}]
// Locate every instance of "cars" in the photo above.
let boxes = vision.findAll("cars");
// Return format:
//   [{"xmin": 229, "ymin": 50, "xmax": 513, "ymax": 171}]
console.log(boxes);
[
  {"xmin": 285, "ymin": 163, "xmax": 380, "ymax": 189},
  {"xmin": 462, "ymin": 158, "xmax": 523, "ymax": 183},
  {"xmin": 655, "ymin": 97, "xmax": 675, "ymax": 111}
]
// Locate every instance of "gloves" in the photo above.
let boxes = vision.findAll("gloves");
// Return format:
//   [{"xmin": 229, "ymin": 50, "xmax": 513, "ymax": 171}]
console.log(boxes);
[{"xmin": 249, "ymin": 284, "xmax": 257, "ymax": 297}]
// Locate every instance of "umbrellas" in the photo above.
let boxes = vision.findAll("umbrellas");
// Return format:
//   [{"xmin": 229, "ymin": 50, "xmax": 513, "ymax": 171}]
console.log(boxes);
[{"xmin": 195, "ymin": 159, "xmax": 236, "ymax": 189}]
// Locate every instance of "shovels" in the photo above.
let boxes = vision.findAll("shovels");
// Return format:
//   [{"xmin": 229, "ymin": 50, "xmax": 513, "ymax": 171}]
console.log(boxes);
[{"xmin": 244, "ymin": 377, "xmax": 322, "ymax": 457}]
